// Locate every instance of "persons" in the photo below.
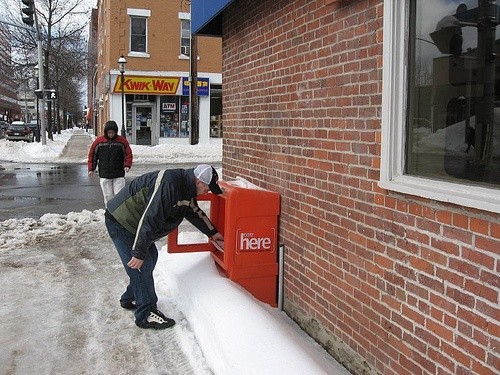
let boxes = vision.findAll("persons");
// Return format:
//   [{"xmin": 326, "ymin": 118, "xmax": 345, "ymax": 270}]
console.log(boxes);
[
  {"xmin": 444, "ymin": 98, "xmax": 476, "ymax": 150},
  {"xmin": 104, "ymin": 164, "xmax": 224, "ymax": 330},
  {"xmin": 88, "ymin": 121, "xmax": 133, "ymax": 211}
]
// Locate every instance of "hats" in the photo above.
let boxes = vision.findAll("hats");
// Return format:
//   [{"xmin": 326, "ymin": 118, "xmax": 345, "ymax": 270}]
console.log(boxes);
[{"xmin": 194, "ymin": 164, "xmax": 223, "ymax": 195}]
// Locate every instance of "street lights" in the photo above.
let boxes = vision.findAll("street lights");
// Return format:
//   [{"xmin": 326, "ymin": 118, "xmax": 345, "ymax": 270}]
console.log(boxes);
[
  {"xmin": 117, "ymin": 52, "xmax": 127, "ymax": 139},
  {"xmin": 34, "ymin": 64, "xmax": 40, "ymax": 142}
]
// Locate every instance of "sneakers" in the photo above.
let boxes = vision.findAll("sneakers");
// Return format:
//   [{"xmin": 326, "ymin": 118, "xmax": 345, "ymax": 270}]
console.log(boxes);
[
  {"xmin": 135, "ymin": 309, "xmax": 175, "ymax": 330},
  {"xmin": 121, "ymin": 300, "xmax": 138, "ymax": 309}
]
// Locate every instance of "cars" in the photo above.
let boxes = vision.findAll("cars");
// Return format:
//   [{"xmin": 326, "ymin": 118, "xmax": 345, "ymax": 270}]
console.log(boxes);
[{"xmin": 0, "ymin": 121, "xmax": 38, "ymax": 143}]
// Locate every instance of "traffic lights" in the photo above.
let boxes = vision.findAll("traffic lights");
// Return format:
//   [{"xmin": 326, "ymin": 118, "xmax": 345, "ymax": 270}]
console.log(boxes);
[
  {"xmin": 21, "ymin": 0, "xmax": 35, "ymax": 27},
  {"xmin": 34, "ymin": 90, "xmax": 43, "ymax": 99},
  {"xmin": 46, "ymin": 90, "xmax": 57, "ymax": 100}
]
